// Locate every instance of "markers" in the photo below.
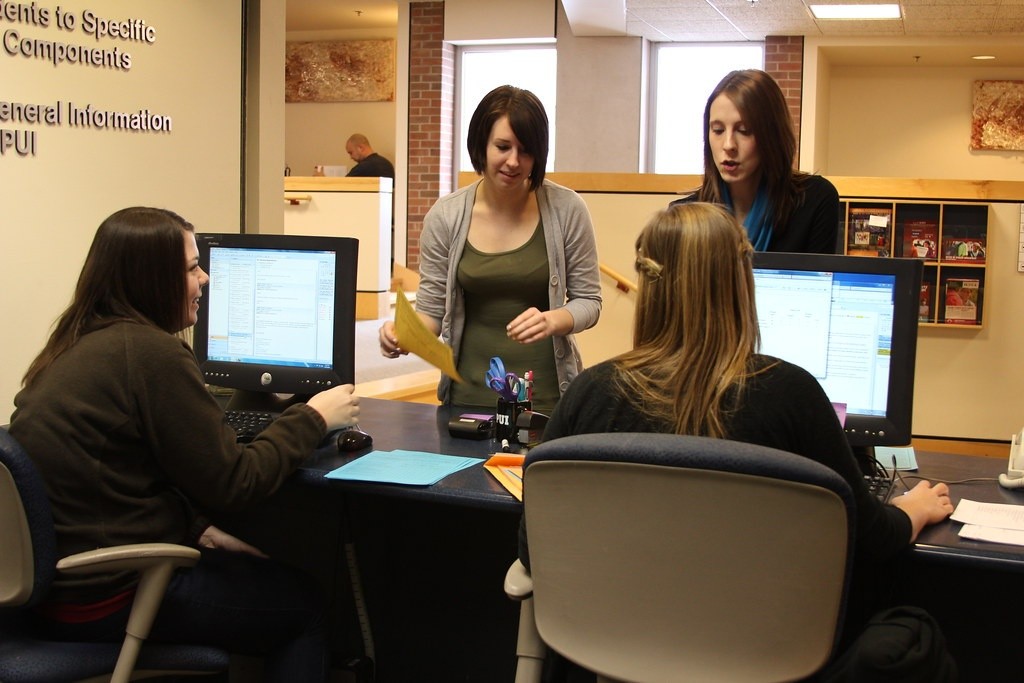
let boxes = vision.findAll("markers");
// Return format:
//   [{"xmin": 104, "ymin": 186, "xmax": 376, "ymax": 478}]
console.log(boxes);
[{"xmin": 502, "ymin": 439, "xmax": 510, "ymax": 453}]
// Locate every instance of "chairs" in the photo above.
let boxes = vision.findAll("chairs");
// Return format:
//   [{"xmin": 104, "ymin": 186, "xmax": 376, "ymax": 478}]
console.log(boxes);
[
  {"xmin": 0, "ymin": 423, "xmax": 232, "ymax": 683},
  {"xmin": 505, "ymin": 432, "xmax": 860, "ymax": 683}
]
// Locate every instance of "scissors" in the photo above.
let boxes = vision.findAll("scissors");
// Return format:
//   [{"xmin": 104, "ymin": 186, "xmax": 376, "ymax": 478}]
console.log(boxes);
[
  {"xmin": 485, "ymin": 357, "xmax": 506, "ymax": 392},
  {"xmin": 490, "ymin": 373, "xmax": 521, "ymax": 403}
]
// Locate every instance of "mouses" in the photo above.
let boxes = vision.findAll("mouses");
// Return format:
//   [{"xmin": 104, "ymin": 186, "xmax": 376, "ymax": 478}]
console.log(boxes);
[{"xmin": 337, "ymin": 430, "xmax": 373, "ymax": 453}]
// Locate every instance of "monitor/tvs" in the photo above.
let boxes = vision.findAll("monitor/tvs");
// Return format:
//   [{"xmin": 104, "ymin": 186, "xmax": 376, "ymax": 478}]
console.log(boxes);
[
  {"xmin": 749, "ymin": 253, "xmax": 926, "ymax": 476},
  {"xmin": 193, "ymin": 233, "xmax": 359, "ymax": 414}
]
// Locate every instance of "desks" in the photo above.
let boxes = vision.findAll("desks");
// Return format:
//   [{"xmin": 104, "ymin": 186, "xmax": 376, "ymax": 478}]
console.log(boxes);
[{"xmin": 206, "ymin": 395, "xmax": 1024, "ymax": 683}]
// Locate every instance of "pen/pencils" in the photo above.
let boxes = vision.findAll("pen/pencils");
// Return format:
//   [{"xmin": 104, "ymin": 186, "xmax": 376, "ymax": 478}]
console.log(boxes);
[{"xmin": 524, "ymin": 371, "xmax": 534, "ymax": 402}]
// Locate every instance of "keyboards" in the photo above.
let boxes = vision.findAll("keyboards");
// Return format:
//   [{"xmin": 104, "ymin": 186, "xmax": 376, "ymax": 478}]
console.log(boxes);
[
  {"xmin": 223, "ymin": 409, "xmax": 275, "ymax": 440},
  {"xmin": 860, "ymin": 473, "xmax": 895, "ymax": 506}
]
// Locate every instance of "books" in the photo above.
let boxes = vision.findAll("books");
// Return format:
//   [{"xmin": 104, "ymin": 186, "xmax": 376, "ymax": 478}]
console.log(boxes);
[
  {"xmin": 848, "ymin": 207, "xmax": 892, "ymax": 258},
  {"xmin": 902, "ymin": 214, "xmax": 938, "ymax": 262},
  {"xmin": 944, "ymin": 277, "xmax": 980, "ymax": 325},
  {"xmin": 918, "ymin": 281, "xmax": 932, "ymax": 323}
]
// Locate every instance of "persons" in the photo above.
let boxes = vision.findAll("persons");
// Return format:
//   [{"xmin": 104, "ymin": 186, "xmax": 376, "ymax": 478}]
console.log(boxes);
[
  {"xmin": 380, "ymin": 85, "xmax": 603, "ymax": 415},
  {"xmin": 669, "ymin": 70, "xmax": 839, "ymax": 254},
  {"xmin": 345, "ymin": 133, "xmax": 395, "ymax": 189},
  {"xmin": 9, "ymin": 207, "xmax": 360, "ymax": 683},
  {"xmin": 520, "ymin": 202, "xmax": 953, "ymax": 661}
]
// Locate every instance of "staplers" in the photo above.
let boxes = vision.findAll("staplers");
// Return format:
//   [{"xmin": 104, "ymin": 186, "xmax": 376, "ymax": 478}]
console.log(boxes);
[{"xmin": 516, "ymin": 412, "xmax": 550, "ymax": 443}]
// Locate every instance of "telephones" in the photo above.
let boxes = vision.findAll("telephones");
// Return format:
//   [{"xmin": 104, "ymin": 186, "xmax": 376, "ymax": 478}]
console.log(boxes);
[{"xmin": 1007, "ymin": 426, "xmax": 1024, "ymax": 480}]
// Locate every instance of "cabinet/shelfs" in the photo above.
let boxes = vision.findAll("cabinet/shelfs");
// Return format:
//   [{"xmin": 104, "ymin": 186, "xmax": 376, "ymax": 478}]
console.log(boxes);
[{"xmin": 836, "ymin": 198, "xmax": 992, "ymax": 330}]
